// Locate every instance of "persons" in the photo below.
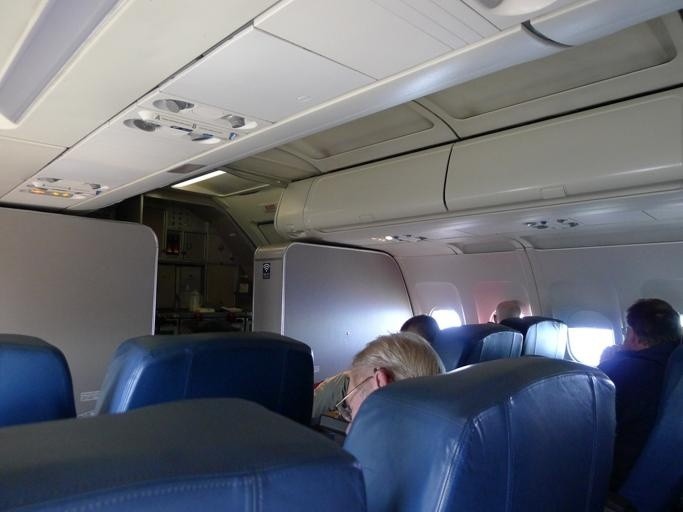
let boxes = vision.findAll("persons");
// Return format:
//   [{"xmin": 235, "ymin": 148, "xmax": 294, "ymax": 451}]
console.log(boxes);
[
  {"xmin": 597, "ymin": 298, "xmax": 683, "ymax": 511},
  {"xmin": 490, "ymin": 299, "xmax": 521, "ymax": 331},
  {"xmin": 399, "ymin": 314, "xmax": 441, "ymax": 345},
  {"xmin": 335, "ymin": 331, "xmax": 446, "ymax": 435}
]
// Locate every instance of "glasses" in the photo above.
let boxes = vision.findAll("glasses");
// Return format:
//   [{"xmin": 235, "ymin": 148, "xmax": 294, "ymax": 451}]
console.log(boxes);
[{"xmin": 336, "ymin": 375, "xmax": 376, "ymax": 423}]
